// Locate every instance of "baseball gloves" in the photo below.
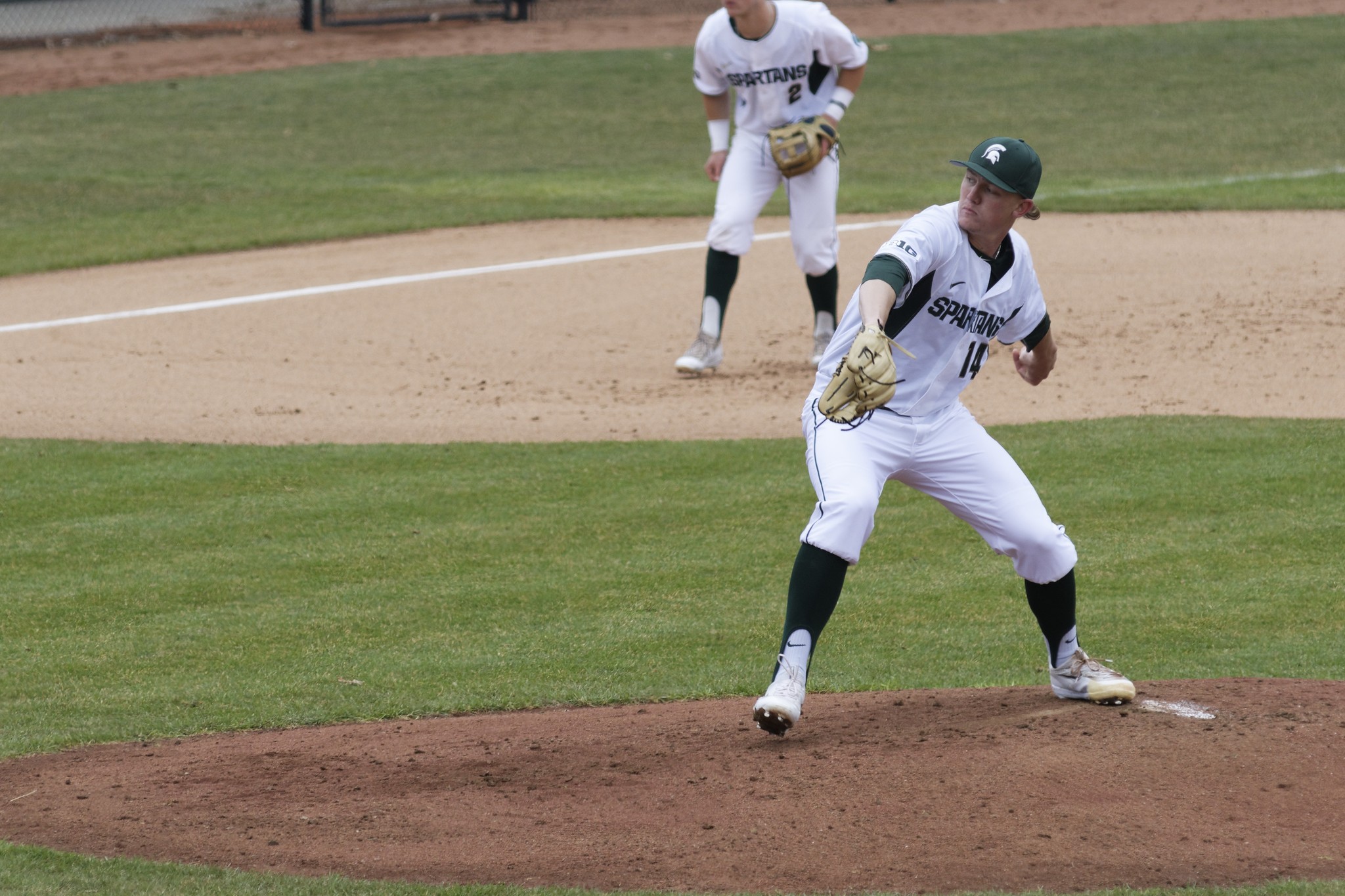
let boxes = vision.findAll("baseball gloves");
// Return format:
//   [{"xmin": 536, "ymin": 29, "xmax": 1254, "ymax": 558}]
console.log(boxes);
[
  {"xmin": 768, "ymin": 119, "xmax": 840, "ymax": 176},
  {"xmin": 818, "ymin": 334, "xmax": 895, "ymax": 426}
]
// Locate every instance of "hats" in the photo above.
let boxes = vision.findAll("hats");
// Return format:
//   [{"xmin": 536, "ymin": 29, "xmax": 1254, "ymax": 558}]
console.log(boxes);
[{"xmin": 950, "ymin": 136, "xmax": 1041, "ymax": 200}]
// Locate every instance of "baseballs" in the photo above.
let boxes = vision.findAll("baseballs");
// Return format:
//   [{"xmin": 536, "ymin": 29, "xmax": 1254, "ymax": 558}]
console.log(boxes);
[{"xmin": 1020, "ymin": 345, "xmax": 1033, "ymax": 366}]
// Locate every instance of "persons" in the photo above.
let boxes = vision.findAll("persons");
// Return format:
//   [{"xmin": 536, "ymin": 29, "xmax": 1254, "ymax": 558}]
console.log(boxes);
[
  {"xmin": 750, "ymin": 136, "xmax": 1136, "ymax": 736},
  {"xmin": 673, "ymin": 0, "xmax": 870, "ymax": 374}
]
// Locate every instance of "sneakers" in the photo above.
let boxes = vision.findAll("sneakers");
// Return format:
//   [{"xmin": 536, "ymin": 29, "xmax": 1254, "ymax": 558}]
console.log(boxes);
[
  {"xmin": 674, "ymin": 337, "xmax": 721, "ymax": 374},
  {"xmin": 1048, "ymin": 649, "xmax": 1136, "ymax": 703},
  {"xmin": 750, "ymin": 653, "xmax": 808, "ymax": 736},
  {"xmin": 810, "ymin": 335, "xmax": 831, "ymax": 365}
]
